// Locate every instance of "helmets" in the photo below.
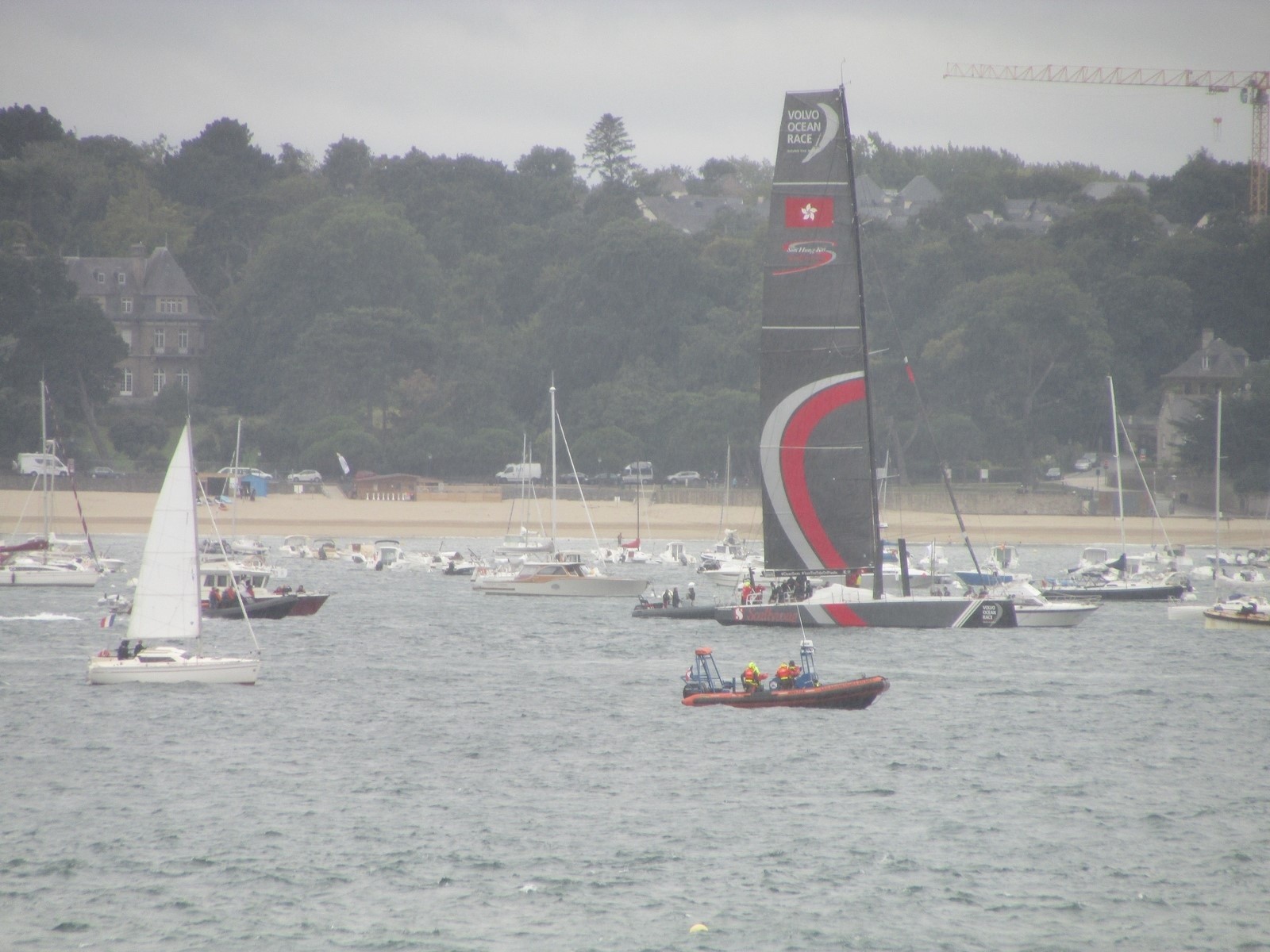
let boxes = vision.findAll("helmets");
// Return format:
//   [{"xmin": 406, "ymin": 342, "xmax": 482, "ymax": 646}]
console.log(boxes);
[
  {"xmin": 689, "ymin": 582, "xmax": 695, "ymax": 587},
  {"xmin": 780, "ymin": 662, "xmax": 787, "ymax": 668},
  {"xmin": 746, "ymin": 582, "xmax": 750, "ymax": 586},
  {"xmin": 748, "ymin": 662, "xmax": 755, "ymax": 668}
]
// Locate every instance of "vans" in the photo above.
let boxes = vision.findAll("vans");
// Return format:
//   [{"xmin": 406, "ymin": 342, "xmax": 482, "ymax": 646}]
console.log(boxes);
[
  {"xmin": 12, "ymin": 452, "xmax": 71, "ymax": 476},
  {"xmin": 617, "ymin": 462, "xmax": 654, "ymax": 485}
]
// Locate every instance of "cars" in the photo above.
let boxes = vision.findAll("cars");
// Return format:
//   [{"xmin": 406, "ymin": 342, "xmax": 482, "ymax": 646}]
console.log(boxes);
[
  {"xmin": 1047, "ymin": 467, "xmax": 1062, "ymax": 479},
  {"xmin": 585, "ymin": 472, "xmax": 624, "ymax": 485},
  {"xmin": 666, "ymin": 471, "xmax": 703, "ymax": 485},
  {"xmin": 557, "ymin": 472, "xmax": 589, "ymax": 485},
  {"xmin": 1074, "ymin": 458, "xmax": 1093, "ymax": 469},
  {"xmin": 287, "ymin": 470, "xmax": 322, "ymax": 482},
  {"xmin": 1083, "ymin": 451, "xmax": 1100, "ymax": 467},
  {"xmin": 88, "ymin": 466, "xmax": 125, "ymax": 478}
]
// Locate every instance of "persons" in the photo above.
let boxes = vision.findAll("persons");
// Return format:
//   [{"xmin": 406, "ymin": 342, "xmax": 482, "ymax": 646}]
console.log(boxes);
[
  {"xmin": 197, "ymin": 484, "xmax": 257, "ymax": 504},
  {"xmin": 617, "ymin": 532, "xmax": 863, "ymax": 693},
  {"xmin": 933, "ymin": 585, "xmax": 988, "ymax": 599},
  {"xmin": 1118, "ymin": 552, "xmax": 1128, "ymax": 581},
  {"xmin": 209, "ymin": 577, "xmax": 305, "ymax": 609},
  {"xmin": 117, "ymin": 640, "xmax": 146, "ymax": 660}
]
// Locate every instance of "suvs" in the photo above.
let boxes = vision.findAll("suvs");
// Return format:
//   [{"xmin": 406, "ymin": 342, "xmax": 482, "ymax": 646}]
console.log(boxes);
[{"xmin": 216, "ymin": 467, "xmax": 273, "ymax": 480}]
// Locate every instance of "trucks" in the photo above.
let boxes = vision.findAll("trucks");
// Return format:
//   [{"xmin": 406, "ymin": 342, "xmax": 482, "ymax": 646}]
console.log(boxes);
[{"xmin": 494, "ymin": 462, "xmax": 542, "ymax": 486}]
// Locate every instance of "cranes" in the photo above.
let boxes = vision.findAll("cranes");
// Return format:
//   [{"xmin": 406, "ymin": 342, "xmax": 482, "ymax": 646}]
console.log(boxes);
[{"xmin": 943, "ymin": 64, "xmax": 1269, "ymax": 227}]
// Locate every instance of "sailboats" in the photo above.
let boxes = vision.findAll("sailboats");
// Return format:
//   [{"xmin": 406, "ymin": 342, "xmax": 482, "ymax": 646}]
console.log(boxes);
[
  {"xmin": 87, "ymin": 414, "xmax": 264, "ymax": 685},
  {"xmin": 491, "ymin": 433, "xmax": 554, "ymax": 553},
  {"xmin": 714, "ymin": 85, "xmax": 1017, "ymax": 634},
  {"xmin": 195, "ymin": 418, "xmax": 494, "ymax": 618},
  {"xmin": 611, "ymin": 371, "xmax": 1268, "ymax": 637},
  {"xmin": 473, "ymin": 369, "xmax": 651, "ymax": 598},
  {"xmin": 1, "ymin": 377, "xmax": 124, "ymax": 586}
]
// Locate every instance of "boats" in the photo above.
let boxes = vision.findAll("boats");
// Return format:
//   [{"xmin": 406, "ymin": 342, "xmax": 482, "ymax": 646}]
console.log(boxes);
[{"xmin": 680, "ymin": 598, "xmax": 891, "ymax": 709}]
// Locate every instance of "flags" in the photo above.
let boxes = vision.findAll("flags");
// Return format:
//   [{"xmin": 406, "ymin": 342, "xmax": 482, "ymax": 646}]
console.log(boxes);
[{"xmin": 101, "ymin": 613, "xmax": 116, "ymax": 628}]
